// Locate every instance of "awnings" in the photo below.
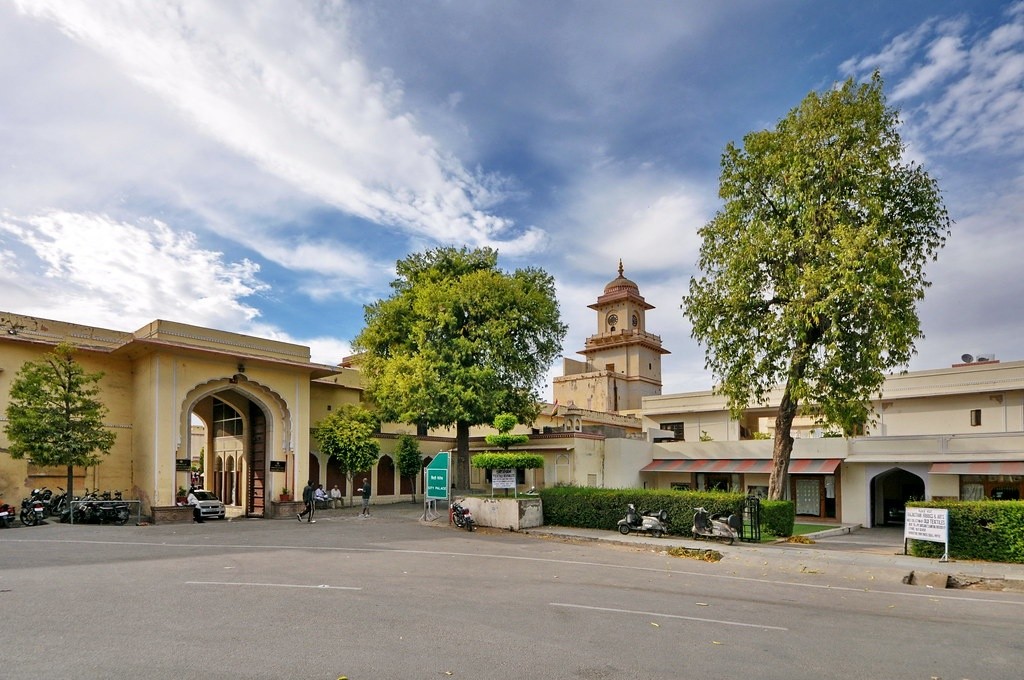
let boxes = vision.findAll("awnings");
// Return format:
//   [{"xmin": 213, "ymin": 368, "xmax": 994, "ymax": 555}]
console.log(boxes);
[
  {"xmin": 639, "ymin": 459, "xmax": 843, "ymax": 474},
  {"xmin": 927, "ymin": 463, "xmax": 1024, "ymax": 475}
]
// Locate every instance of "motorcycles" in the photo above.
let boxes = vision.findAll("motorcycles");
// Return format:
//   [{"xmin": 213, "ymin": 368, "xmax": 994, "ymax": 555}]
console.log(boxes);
[{"xmin": 20, "ymin": 486, "xmax": 130, "ymax": 527}]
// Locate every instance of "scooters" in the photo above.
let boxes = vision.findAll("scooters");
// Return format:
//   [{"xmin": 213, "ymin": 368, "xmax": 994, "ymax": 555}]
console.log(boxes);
[
  {"xmin": 450, "ymin": 499, "xmax": 474, "ymax": 533},
  {"xmin": 616, "ymin": 503, "xmax": 668, "ymax": 539},
  {"xmin": 0, "ymin": 504, "xmax": 15, "ymax": 528},
  {"xmin": 691, "ymin": 507, "xmax": 743, "ymax": 546}
]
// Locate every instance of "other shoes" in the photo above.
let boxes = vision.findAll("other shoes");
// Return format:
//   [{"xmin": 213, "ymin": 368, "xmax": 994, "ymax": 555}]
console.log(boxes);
[
  {"xmin": 297, "ymin": 513, "xmax": 302, "ymax": 522},
  {"xmin": 308, "ymin": 520, "xmax": 316, "ymax": 524},
  {"xmin": 359, "ymin": 514, "xmax": 364, "ymax": 517},
  {"xmin": 365, "ymin": 514, "xmax": 370, "ymax": 518}
]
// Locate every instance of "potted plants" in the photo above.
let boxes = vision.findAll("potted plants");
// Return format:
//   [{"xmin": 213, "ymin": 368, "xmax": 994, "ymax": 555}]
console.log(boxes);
[
  {"xmin": 176, "ymin": 486, "xmax": 187, "ymax": 504},
  {"xmin": 279, "ymin": 487, "xmax": 290, "ymax": 502}
]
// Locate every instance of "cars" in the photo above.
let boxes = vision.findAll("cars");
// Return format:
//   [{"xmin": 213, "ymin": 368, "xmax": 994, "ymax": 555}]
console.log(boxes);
[{"xmin": 193, "ymin": 490, "xmax": 226, "ymax": 520}]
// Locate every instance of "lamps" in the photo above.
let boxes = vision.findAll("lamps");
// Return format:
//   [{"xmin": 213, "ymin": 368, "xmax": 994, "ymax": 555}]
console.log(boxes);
[{"xmin": 8, "ymin": 329, "xmax": 17, "ymax": 335}]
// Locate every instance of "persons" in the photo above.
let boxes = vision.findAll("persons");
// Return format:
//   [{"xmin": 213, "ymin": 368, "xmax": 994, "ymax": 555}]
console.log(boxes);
[
  {"xmin": 314, "ymin": 485, "xmax": 328, "ymax": 510},
  {"xmin": 296, "ymin": 480, "xmax": 316, "ymax": 523},
  {"xmin": 331, "ymin": 485, "xmax": 345, "ymax": 509},
  {"xmin": 186, "ymin": 483, "xmax": 206, "ymax": 524},
  {"xmin": 357, "ymin": 478, "xmax": 371, "ymax": 518}
]
